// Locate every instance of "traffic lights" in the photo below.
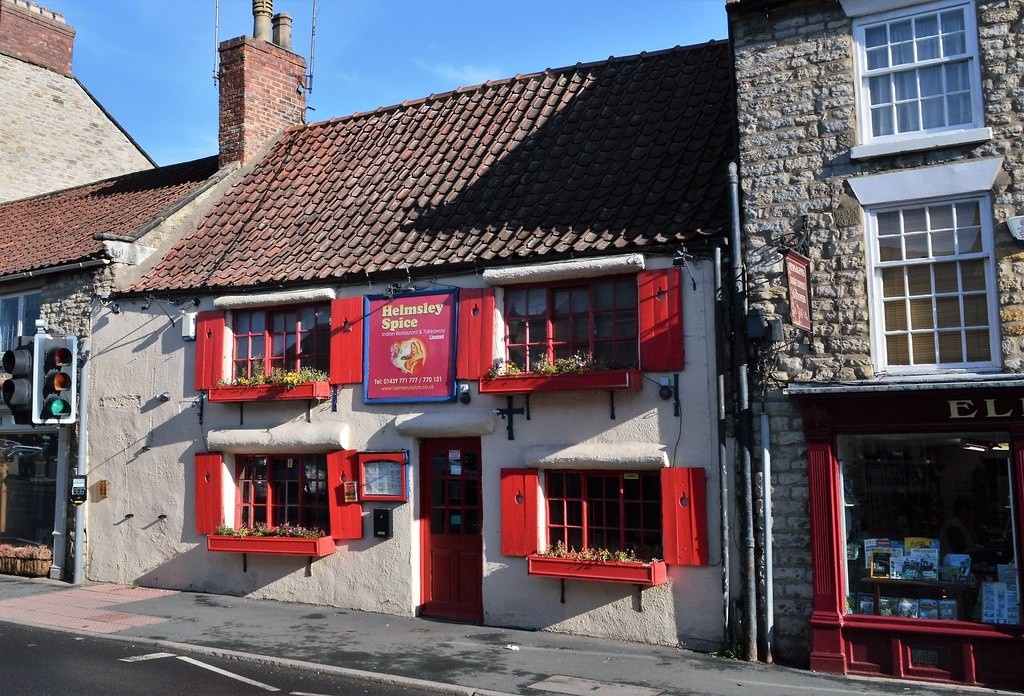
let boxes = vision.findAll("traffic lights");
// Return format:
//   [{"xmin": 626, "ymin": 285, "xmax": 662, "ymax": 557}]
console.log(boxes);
[{"xmin": 2, "ymin": 335, "xmax": 77, "ymax": 426}]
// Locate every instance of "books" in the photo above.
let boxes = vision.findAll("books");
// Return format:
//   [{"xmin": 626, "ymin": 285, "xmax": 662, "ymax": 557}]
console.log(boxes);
[{"xmin": 845, "ymin": 535, "xmax": 1020, "ymax": 626}]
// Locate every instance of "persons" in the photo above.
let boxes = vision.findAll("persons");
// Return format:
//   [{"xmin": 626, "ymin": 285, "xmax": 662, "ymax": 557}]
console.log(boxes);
[{"xmin": 948, "ymin": 496, "xmax": 984, "ymax": 556}]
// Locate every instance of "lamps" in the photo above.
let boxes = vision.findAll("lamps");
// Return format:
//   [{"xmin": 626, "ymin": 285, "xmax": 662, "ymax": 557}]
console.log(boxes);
[{"xmin": 960, "ymin": 439, "xmax": 1002, "ymax": 452}]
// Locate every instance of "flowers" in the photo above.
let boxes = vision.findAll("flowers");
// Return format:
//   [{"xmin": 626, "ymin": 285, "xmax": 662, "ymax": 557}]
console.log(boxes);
[
  {"xmin": 215, "ymin": 352, "xmax": 331, "ymax": 391},
  {"xmin": 489, "ymin": 348, "xmax": 629, "ymax": 381},
  {"xmin": 217, "ymin": 522, "xmax": 326, "ymax": 539}
]
